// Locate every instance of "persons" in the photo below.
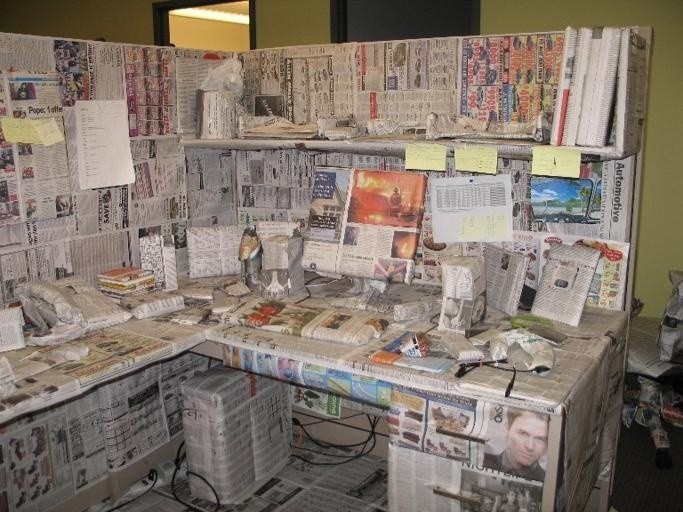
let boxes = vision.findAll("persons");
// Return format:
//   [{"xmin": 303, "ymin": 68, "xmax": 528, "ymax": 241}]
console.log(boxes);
[{"xmin": 483, "ymin": 406, "xmax": 550, "ymax": 483}]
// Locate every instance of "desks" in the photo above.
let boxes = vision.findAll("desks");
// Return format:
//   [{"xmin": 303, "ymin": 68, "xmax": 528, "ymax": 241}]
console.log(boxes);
[{"xmin": 1, "ymin": 24, "xmax": 656, "ymax": 512}]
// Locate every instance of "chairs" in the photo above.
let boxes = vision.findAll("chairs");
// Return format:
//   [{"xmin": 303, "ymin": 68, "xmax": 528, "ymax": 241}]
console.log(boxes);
[{"xmin": 614, "ymin": 267, "xmax": 683, "ymax": 470}]
